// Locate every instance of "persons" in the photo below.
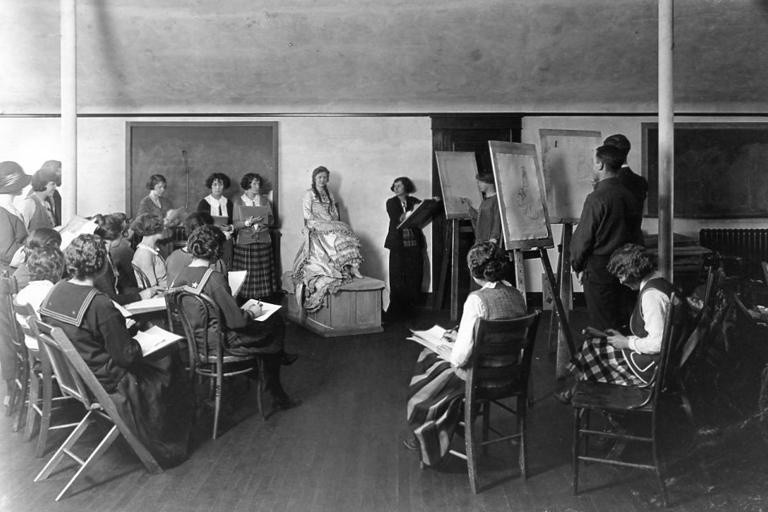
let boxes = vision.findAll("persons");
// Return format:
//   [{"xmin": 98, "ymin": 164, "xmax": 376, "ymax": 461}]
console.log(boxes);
[
  {"xmin": 40, "ymin": 233, "xmax": 195, "ymax": 466},
  {"xmin": 603, "ymin": 134, "xmax": 648, "ymax": 218},
  {"xmin": 169, "ymin": 225, "xmax": 299, "ymax": 411},
  {"xmin": 196, "ymin": 173, "xmax": 234, "ymax": 273},
  {"xmin": 39, "ymin": 160, "xmax": 62, "ymax": 227},
  {"xmin": 231, "ymin": 173, "xmax": 278, "ymax": 301},
  {"xmin": 405, "ymin": 240, "xmax": 525, "ymax": 468},
  {"xmin": 20, "ymin": 168, "xmax": 59, "ymax": 234},
  {"xmin": 290, "ymin": 165, "xmax": 364, "ymax": 315},
  {"xmin": 14, "ymin": 229, "xmax": 62, "ymax": 290},
  {"xmin": 381, "ymin": 177, "xmax": 427, "ymax": 321},
  {"xmin": 20, "ymin": 168, "xmax": 59, "ymax": 467},
  {"xmin": 459, "ymin": 171, "xmax": 503, "ymax": 244},
  {"xmin": 136, "ymin": 174, "xmax": 174, "ymax": 220},
  {"xmin": 93, "ymin": 214, "xmax": 228, "ymax": 289},
  {"xmin": 569, "ymin": 145, "xmax": 642, "ymax": 333},
  {"xmin": 15, "ymin": 246, "xmax": 65, "ymax": 360},
  {"xmin": 0, "ymin": 161, "xmax": 35, "ymax": 410},
  {"xmin": 550, "ymin": 242, "xmax": 680, "ymax": 453}
]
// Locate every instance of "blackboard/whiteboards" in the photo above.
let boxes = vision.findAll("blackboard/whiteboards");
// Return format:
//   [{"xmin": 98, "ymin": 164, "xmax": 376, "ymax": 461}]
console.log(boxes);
[
  {"xmin": 124, "ymin": 121, "xmax": 278, "ymax": 231},
  {"xmin": 641, "ymin": 123, "xmax": 768, "ymax": 219}
]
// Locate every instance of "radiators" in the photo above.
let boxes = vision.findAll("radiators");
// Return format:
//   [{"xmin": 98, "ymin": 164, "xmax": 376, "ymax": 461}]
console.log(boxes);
[{"xmin": 699, "ymin": 226, "xmax": 768, "ymax": 282}]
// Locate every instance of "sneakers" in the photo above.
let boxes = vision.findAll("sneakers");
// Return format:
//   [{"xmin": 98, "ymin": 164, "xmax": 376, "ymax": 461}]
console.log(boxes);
[
  {"xmin": 403, "ymin": 438, "xmax": 422, "ymax": 450},
  {"xmin": 552, "ymin": 390, "xmax": 573, "ymax": 405}
]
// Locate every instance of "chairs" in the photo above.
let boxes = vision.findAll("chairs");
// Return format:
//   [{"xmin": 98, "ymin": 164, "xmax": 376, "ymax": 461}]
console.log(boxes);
[
  {"xmin": 11, "ymin": 298, "xmax": 164, "ymax": 501},
  {"xmin": 2, "ymin": 250, "xmax": 277, "ymax": 458},
  {"xmin": 571, "ymin": 291, "xmax": 691, "ymax": 507},
  {"xmin": 443, "ymin": 309, "xmax": 539, "ymax": 495},
  {"xmin": 677, "ymin": 256, "xmax": 765, "ymax": 436}
]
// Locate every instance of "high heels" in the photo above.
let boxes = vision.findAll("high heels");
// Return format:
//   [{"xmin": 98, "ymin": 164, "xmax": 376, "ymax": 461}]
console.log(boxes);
[
  {"xmin": 280, "ymin": 352, "xmax": 298, "ymax": 365},
  {"xmin": 270, "ymin": 394, "xmax": 304, "ymax": 411}
]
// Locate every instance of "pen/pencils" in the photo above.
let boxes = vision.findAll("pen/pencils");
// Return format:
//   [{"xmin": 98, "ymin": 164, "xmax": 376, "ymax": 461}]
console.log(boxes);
[{"xmin": 257, "ymin": 299, "xmax": 261, "ymax": 304}]
